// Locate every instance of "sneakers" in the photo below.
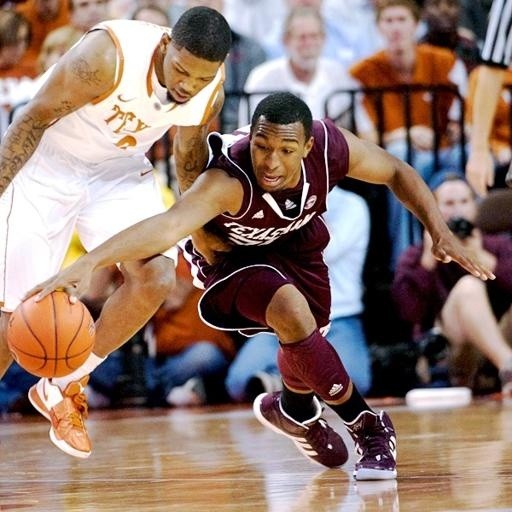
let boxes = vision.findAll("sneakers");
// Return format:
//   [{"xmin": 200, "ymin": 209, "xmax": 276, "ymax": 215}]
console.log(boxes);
[
  {"xmin": 343, "ymin": 410, "xmax": 398, "ymax": 481},
  {"xmin": 27, "ymin": 374, "xmax": 93, "ymax": 459},
  {"xmin": 252, "ymin": 391, "xmax": 348, "ymax": 468}
]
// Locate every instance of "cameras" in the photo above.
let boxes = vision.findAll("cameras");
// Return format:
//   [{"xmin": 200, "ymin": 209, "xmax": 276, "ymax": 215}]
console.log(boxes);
[{"xmin": 446, "ymin": 216, "xmax": 475, "ymax": 239}]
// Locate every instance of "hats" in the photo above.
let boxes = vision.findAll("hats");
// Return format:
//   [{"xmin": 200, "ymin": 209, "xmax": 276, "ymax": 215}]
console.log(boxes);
[{"xmin": 427, "ymin": 167, "xmax": 464, "ymax": 193}]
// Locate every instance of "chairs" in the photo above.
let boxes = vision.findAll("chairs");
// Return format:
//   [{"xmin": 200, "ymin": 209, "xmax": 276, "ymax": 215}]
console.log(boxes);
[{"xmin": 222, "ymin": 36, "xmax": 268, "ymax": 135}]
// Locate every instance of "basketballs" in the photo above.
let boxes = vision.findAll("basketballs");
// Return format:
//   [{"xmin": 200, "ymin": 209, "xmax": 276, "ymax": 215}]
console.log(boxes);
[{"xmin": 7, "ymin": 289, "xmax": 97, "ymax": 378}]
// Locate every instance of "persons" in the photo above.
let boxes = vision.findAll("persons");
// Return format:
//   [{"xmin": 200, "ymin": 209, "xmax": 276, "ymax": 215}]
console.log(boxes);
[
  {"xmin": 1, "ymin": 0, "xmax": 511, "ymax": 415},
  {"xmin": 0, "ymin": 5, "xmax": 233, "ymax": 459},
  {"xmin": 22, "ymin": 92, "xmax": 496, "ymax": 480}
]
